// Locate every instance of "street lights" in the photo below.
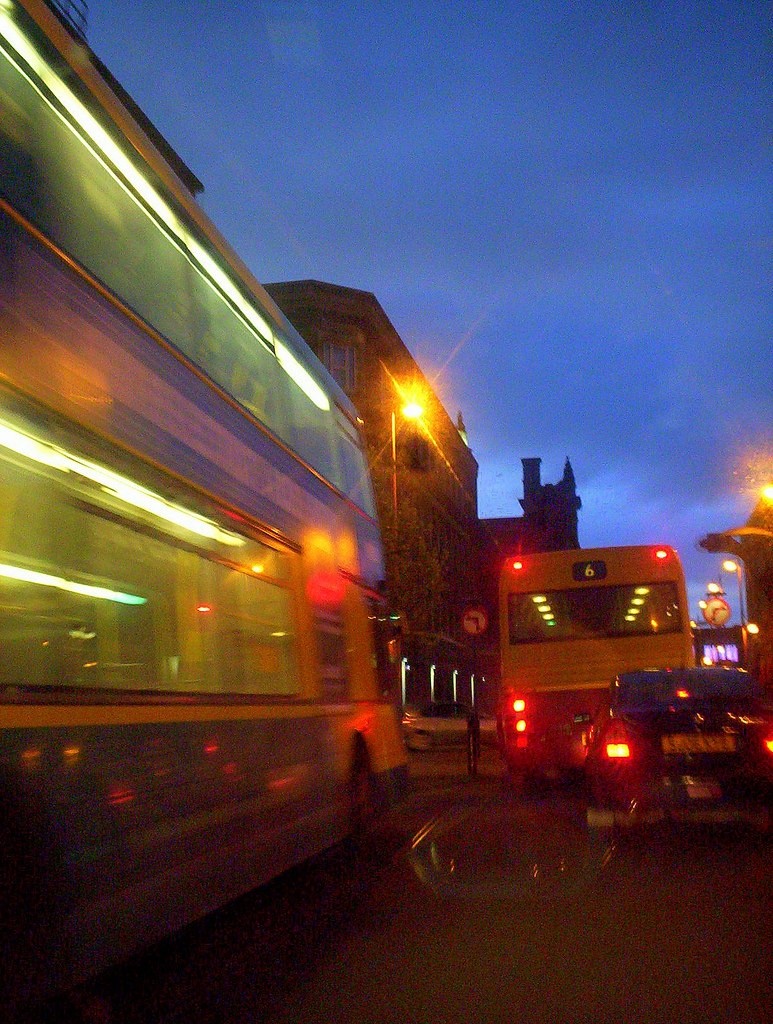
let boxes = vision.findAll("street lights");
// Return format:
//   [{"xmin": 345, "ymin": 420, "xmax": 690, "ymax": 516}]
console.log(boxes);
[
  {"xmin": 389, "ymin": 400, "xmax": 422, "ymax": 590},
  {"xmin": 470, "ymin": 673, "xmax": 478, "ymax": 707},
  {"xmin": 402, "ymin": 657, "xmax": 407, "ymax": 705},
  {"xmin": 453, "ymin": 668, "xmax": 458, "ymax": 702},
  {"xmin": 724, "ymin": 559, "xmax": 746, "ymax": 627},
  {"xmin": 430, "ymin": 664, "xmax": 437, "ymax": 703}
]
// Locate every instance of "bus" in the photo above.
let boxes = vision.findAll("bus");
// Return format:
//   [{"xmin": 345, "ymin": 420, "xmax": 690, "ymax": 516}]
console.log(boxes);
[
  {"xmin": 0, "ymin": 0, "xmax": 415, "ymax": 1023},
  {"xmin": 499, "ymin": 545, "xmax": 696, "ymax": 796}
]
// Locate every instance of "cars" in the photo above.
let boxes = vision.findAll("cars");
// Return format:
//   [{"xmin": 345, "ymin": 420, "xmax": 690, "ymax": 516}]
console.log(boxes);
[
  {"xmin": 574, "ymin": 664, "xmax": 773, "ymax": 827},
  {"xmin": 399, "ymin": 701, "xmax": 504, "ymax": 751}
]
